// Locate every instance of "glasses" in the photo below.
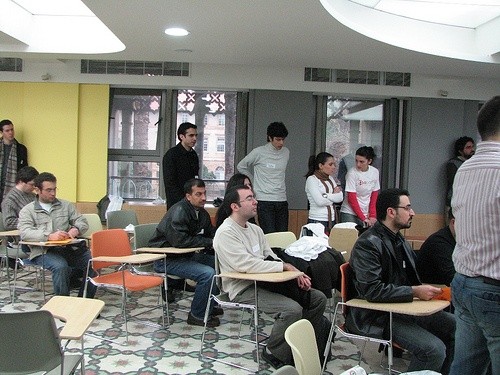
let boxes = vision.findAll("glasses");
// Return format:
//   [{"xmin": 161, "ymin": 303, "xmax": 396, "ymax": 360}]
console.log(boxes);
[
  {"xmin": 234, "ymin": 195, "xmax": 257, "ymax": 203},
  {"xmin": 390, "ymin": 204, "xmax": 411, "ymax": 211},
  {"xmin": 39, "ymin": 185, "xmax": 59, "ymax": 193}
]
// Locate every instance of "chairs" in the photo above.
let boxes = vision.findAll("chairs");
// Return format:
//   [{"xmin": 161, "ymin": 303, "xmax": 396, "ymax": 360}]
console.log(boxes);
[
  {"xmin": 107, "ymin": 210, "xmax": 139, "ymax": 229},
  {"xmin": 322, "ymin": 262, "xmax": 449, "ymax": 375},
  {"xmin": 0, "ymin": 213, "xmax": 80, "ymax": 306},
  {"xmin": 77, "ymin": 214, "xmax": 104, "ymax": 238},
  {"xmin": 133, "ymin": 223, "xmax": 204, "ymax": 326},
  {"xmin": 328, "ymin": 228, "xmax": 359, "ymax": 249},
  {"xmin": 0, "ymin": 296, "xmax": 106, "ymax": 375},
  {"xmin": 83, "ymin": 229, "xmax": 166, "ymax": 346},
  {"xmin": 200, "ymin": 252, "xmax": 304, "ymax": 373},
  {"xmin": 264, "ymin": 232, "xmax": 297, "ymax": 248},
  {"xmin": 284, "ymin": 320, "xmax": 322, "ymax": 375}
]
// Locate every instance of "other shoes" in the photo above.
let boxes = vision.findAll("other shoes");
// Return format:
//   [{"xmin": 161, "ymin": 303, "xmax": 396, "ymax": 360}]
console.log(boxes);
[
  {"xmin": 206, "ymin": 307, "xmax": 223, "ymax": 316},
  {"xmin": 187, "ymin": 312, "xmax": 221, "ymax": 326},
  {"xmin": 180, "ymin": 283, "xmax": 195, "ymax": 292},
  {"xmin": 260, "ymin": 346, "xmax": 284, "ymax": 369},
  {"xmin": 161, "ymin": 285, "xmax": 175, "ymax": 304},
  {"xmin": 69, "ymin": 279, "xmax": 82, "ymax": 289}
]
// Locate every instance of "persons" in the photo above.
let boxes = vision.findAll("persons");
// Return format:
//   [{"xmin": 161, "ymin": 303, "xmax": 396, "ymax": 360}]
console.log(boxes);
[
  {"xmin": 304, "ymin": 151, "xmax": 343, "ymax": 237},
  {"xmin": 345, "ymin": 187, "xmax": 457, "ymax": 375},
  {"xmin": 162, "ymin": 121, "xmax": 200, "ymax": 302},
  {"xmin": 0, "ymin": 119, "xmax": 28, "ymax": 269},
  {"xmin": 236, "ymin": 121, "xmax": 289, "ymax": 234},
  {"xmin": 450, "ymin": 95, "xmax": 500, "ymax": 375},
  {"xmin": 19, "ymin": 172, "xmax": 101, "ymax": 323},
  {"xmin": 442, "ymin": 135, "xmax": 475, "ymax": 220},
  {"xmin": 212, "ymin": 173, "xmax": 327, "ymax": 370},
  {"xmin": 340, "ymin": 146, "xmax": 381, "ymax": 236},
  {"xmin": 148, "ymin": 178, "xmax": 224, "ymax": 327},
  {"xmin": 1, "ymin": 165, "xmax": 83, "ymax": 290}
]
